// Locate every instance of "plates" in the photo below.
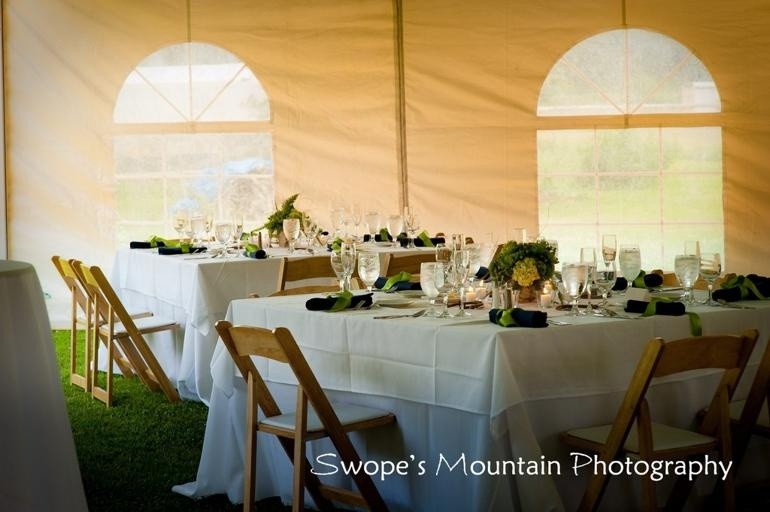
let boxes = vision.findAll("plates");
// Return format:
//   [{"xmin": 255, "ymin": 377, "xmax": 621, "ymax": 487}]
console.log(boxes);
[
  {"xmin": 396, "ymin": 290, "xmax": 425, "ymax": 298},
  {"xmin": 377, "ymin": 298, "xmax": 414, "ymax": 307}
]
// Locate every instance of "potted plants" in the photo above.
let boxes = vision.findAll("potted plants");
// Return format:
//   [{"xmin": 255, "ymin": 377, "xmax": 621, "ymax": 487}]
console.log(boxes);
[
  {"xmin": 251, "ymin": 193, "xmax": 309, "ymax": 248},
  {"xmin": 489, "ymin": 239, "xmax": 560, "ymax": 304}
]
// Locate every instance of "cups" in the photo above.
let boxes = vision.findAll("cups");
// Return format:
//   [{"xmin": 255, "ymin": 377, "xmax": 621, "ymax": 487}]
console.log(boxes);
[
  {"xmin": 476, "ymin": 283, "xmax": 492, "ymax": 301},
  {"xmin": 536, "ymin": 290, "xmax": 558, "ymax": 311},
  {"xmin": 499, "ymin": 290, "xmax": 521, "ymax": 308},
  {"xmin": 559, "ymin": 283, "xmax": 583, "ymax": 306},
  {"xmin": 474, "ymin": 279, "xmax": 487, "ymax": 302}
]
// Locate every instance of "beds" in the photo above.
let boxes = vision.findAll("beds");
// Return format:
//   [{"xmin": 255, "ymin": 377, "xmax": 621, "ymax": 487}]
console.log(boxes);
[{"xmin": 89, "ymin": 243, "xmax": 436, "ymax": 407}]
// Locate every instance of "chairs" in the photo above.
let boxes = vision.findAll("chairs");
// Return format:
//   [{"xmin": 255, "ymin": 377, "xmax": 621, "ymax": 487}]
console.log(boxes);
[
  {"xmin": 697, "ymin": 339, "xmax": 770, "ymax": 512},
  {"xmin": 51, "ymin": 255, "xmax": 153, "ymax": 392},
  {"xmin": 71, "ymin": 259, "xmax": 181, "ymax": 408},
  {"xmin": 564, "ymin": 328, "xmax": 760, "ymax": 512},
  {"xmin": 214, "ymin": 320, "xmax": 396, "ymax": 512}
]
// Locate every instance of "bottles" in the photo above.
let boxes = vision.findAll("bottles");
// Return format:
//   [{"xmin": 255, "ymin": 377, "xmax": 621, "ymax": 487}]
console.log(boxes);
[
  {"xmin": 491, "ymin": 286, "xmax": 499, "ymax": 309},
  {"xmin": 505, "ymin": 287, "xmax": 513, "ymax": 309}
]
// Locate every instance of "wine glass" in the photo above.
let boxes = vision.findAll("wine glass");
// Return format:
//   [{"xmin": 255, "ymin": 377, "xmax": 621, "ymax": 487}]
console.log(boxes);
[
  {"xmin": 403, "ymin": 207, "xmax": 420, "ymax": 248},
  {"xmin": 215, "ymin": 216, "xmax": 231, "ymax": 259},
  {"xmin": 618, "ymin": 242, "xmax": 641, "ymax": 295},
  {"xmin": 329, "ymin": 207, "xmax": 344, "ymax": 239},
  {"xmin": 512, "ymin": 227, "xmax": 527, "ymax": 242},
  {"xmin": 461, "ymin": 243, "xmax": 481, "ymax": 292},
  {"xmin": 592, "ymin": 259, "xmax": 618, "ymax": 306},
  {"xmin": 673, "ymin": 253, "xmax": 700, "ymax": 304},
  {"xmin": 330, "ymin": 250, "xmax": 345, "ymax": 290},
  {"xmin": 601, "ymin": 232, "xmax": 617, "ymax": 297},
  {"xmin": 435, "ymin": 241, "xmax": 454, "ymax": 318},
  {"xmin": 174, "ymin": 214, "xmax": 188, "ymax": 242},
  {"xmin": 192, "ymin": 212, "xmax": 207, "ymax": 248},
  {"xmin": 420, "ymin": 261, "xmax": 446, "ymax": 316},
  {"xmin": 340, "ymin": 241, "xmax": 357, "ymax": 293},
  {"xmin": 232, "ymin": 215, "xmax": 243, "ymax": 258},
  {"xmin": 302, "ymin": 210, "xmax": 319, "ymax": 256},
  {"xmin": 549, "ymin": 271, "xmax": 562, "ymax": 306},
  {"xmin": 435, "ymin": 261, "xmax": 454, "ymax": 318},
  {"xmin": 452, "ymin": 249, "xmax": 473, "ymax": 318},
  {"xmin": 366, "ymin": 213, "xmax": 380, "ymax": 247},
  {"xmin": 184, "ymin": 217, "xmax": 196, "ymax": 241},
  {"xmin": 357, "ymin": 252, "xmax": 381, "ymax": 294},
  {"xmin": 197, "ymin": 212, "xmax": 213, "ymax": 248},
  {"xmin": 579, "ymin": 247, "xmax": 597, "ymax": 315},
  {"xmin": 283, "ymin": 218, "xmax": 300, "ymax": 255},
  {"xmin": 386, "ymin": 214, "xmax": 402, "ymax": 249},
  {"xmin": 449, "ymin": 232, "xmax": 463, "ymax": 249},
  {"xmin": 697, "ymin": 251, "xmax": 723, "ymax": 306},
  {"xmin": 561, "ymin": 260, "xmax": 586, "ymax": 316},
  {"xmin": 349, "ymin": 204, "xmax": 361, "ymax": 240}
]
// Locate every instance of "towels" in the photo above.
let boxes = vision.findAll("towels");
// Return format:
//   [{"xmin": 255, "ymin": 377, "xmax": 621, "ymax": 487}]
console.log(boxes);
[
  {"xmin": 611, "ymin": 270, "xmax": 663, "ymax": 291},
  {"xmin": 624, "ymin": 298, "xmax": 685, "ymax": 317},
  {"xmin": 712, "ymin": 274, "xmax": 770, "ymax": 302},
  {"xmin": 373, "ymin": 276, "xmax": 423, "ymax": 290},
  {"xmin": 475, "ymin": 267, "xmax": 490, "ymax": 279},
  {"xmin": 489, "ymin": 307, "xmax": 547, "ymax": 328},
  {"xmin": 130, "ymin": 241, "xmax": 165, "ymax": 248},
  {"xmin": 362, "ymin": 228, "xmax": 445, "ymax": 249},
  {"xmin": 305, "ymin": 292, "xmax": 374, "ymax": 313},
  {"xmin": 158, "ymin": 247, "xmax": 207, "ymax": 255},
  {"xmin": 242, "ymin": 248, "xmax": 267, "ymax": 259}
]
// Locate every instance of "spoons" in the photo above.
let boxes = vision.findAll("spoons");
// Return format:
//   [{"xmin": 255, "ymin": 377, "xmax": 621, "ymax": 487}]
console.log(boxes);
[{"xmin": 375, "ymin": 308, "xmax": 423, "ymax": 321}]
